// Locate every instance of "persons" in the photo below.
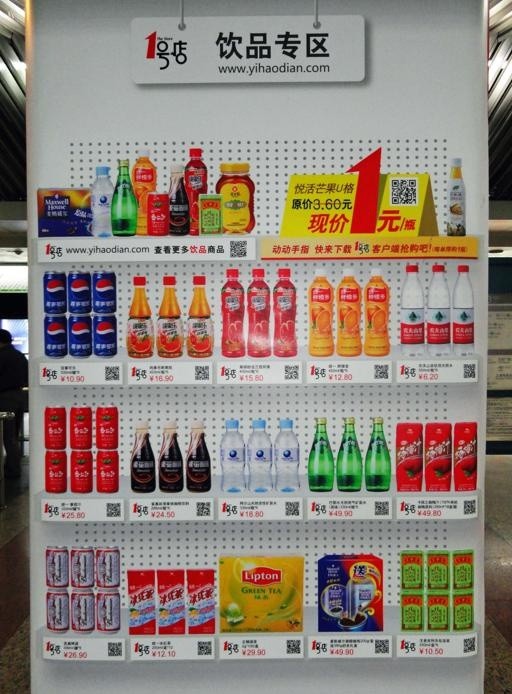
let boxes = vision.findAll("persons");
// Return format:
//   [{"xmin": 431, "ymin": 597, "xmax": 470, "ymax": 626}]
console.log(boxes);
[{"xmin": 1, "ymin": 329, "xmax": 28, "ymax": 467}]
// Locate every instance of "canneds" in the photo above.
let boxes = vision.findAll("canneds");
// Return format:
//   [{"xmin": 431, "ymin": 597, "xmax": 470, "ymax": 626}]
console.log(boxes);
[
  {"xmin": 147, "ymin": 192, "xmax": 170, "ymax": 238},
  {"xmin": 70, "ymin": 406, "xmax": 93, "ymax": 449},
  {"xmin": 97, "ymin": 404, "xmax": 118, "ymax": 450},
  {"xmin": 94, "ymin": 314, "xmax": 117, "ymax": 358},
  {"xmin": 71, "ymin": 589, "xmax": 95, "ymax": 633},
  {"xmin": 44, "ymin": 406, "xmax": 67, "ymax": 450},
  {"xmin": 97, "ymin": 590, "xmax": 121, "ymax": 632},
  {"xmin": 71, "ymin": 546, "xmax": 94, "ymax": 588},
  {"xmin": 46, "ymin": 589, "xmax": 69, "ymax": 633},
  {"xmin": 96, "ymin": 545, "xmax": 121, "ymax": 589},
  {"xmin": 71, "ymin": 270, "xmax": 91, "ymax": 314},
  {"xmin": 45, "ymin": 546, "xmax": 69, "ymax": 587},
  {"xmin": 93, "ymin": 270, "xmax": 117, "ymax": 313},
  {"xmin": 44, "ymin": 270, "xmax": 68, "ymax": 315},
  {"xmin": 44, "ymin": 450, "xmax": 67, "ymax": 494},
  {"xmin": 69, "ymin": 315, "xmax": 92, "ymax": 358},
  {"xmin": 44, "ymin": 314, "xmax": 67, "ymax": 359},
  {"xmin": 96, "ymin": 449, "xmax": 120, "ymax": 493},
  {"xmin": 70, "ymin": 451, "xmax": 94, "ymax": 493}
]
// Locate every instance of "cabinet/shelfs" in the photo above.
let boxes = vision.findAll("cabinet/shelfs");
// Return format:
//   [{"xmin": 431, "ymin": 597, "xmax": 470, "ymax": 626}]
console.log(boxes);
[{"xmin": 26, "ymin": 1, "xmax": 488, "ymax": 691}]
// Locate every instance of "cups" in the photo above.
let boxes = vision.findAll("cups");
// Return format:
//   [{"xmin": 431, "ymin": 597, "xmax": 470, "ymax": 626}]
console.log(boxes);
[
  {"xmin": 329, "ymin": 606, "xmax": 345, "ymax": 614},
  {"xmin": 337, "ymin": 613, "xmax": 368, "ymax": 632}
]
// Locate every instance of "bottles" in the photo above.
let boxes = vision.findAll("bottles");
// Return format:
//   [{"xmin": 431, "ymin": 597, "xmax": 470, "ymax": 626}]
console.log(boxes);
[
  {"xmin": 401, "ymin": 263, "xmax": 476, "ymax": 357},
  {"xmin": 91, "ymin": 149, "xmax": 257, "ymax": 238},
  {"xmin": 446, "ymin": 158, "xmax": 466, "ymax": 235},
  {"xmin": 219, "ymin": 419, "xmax": 301, "ymax": 493},
  {"xmin": 221, "ymin": 268, "xmax": 298, "ymax": 357},
  {"xmin": 310, "ymin": 266, "xmax": 391, "ymax": 356},
  {"xmin": 127, "ymin": 276, "xmax": 214, "ymax": 358},
  {"xmin": 130, "ymin": 419, "xmax": 212, "ymax": 493},
  {"xmin": 308, "ymin": 416, "xmax": 391, "ymax": 492}
]
